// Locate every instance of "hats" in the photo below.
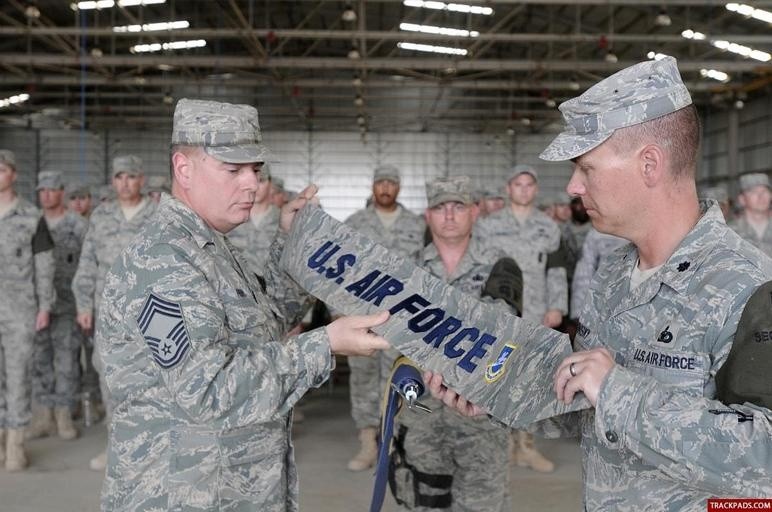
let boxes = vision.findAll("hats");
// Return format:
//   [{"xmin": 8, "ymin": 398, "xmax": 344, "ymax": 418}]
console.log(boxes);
[
  {"xmin": 111, "ymin": 155, "xmax": 145, "ymax": 178},
  {"xmin": 739, "ymin": 173, "xmax": 771, "ymax": 190},
  {"xmin": 540, "ymin": 198, "xmax": 553, "ymax": 207},
  {"xmin": 538, "ymin": 56, "xmax": 694, "ymax": 162},
  {"xmin": 272, "ymin": 176, "xmax": 283, "ymax": 192},
  {"xmin": 374, "ymin": 166, "xmax": 401, "ymax": 185},
  {"xmin": 34, "ymin": 170, "xmax": 64, "ymax": 190},
  {"xmin": 260, "ymin": 165, "xmax": 270, "ymax": 180},
  {"xmin": 0, "ymin": 148, "xmax": 16, "ymax": 170},
  {"xmin": 68, "ymin": 182, "xmax": 90, "ymax": 197},
  {"xmin": 99, "ymin": 185, "xmax": 116, "ymax": 199},
  {"xmin": 171, "ymin": 98, "xmax": 268, "ymax": 163},
  {"xmin": 146, "ymin": 175, "xmax": 170, "ymax": 192},
  {"xmin": 554, "ymin": 192, "xmax": 571, "ymax": 204},
  {"xmin": 484, "ymin": 187, "xmax": 505, "ymax": 198},
  {"xmin": 471, "ymin": 189, "xmax": 483, "ymax": 203},
  {"xmin": 702, "ymin": 188, "xmax": 728, "ymax": 202},
  {"xmin": 507, "ymin": 165, "xmax": 538, "ymax": 182},
  {"xmin": 425, "ymin": 176, "xmax": 471, "ymax": 207}
]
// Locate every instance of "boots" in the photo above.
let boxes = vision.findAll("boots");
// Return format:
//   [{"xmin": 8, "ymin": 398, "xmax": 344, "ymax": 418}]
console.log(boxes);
[
  {"xmin": 1, "ymin": 429, "xmax": 7, "ymax": 467},
  {"xmin": 55, "ymin": 406, "xmax": 76, "ymax": 439},
  {"xmin": 347, "ymin": 428, "xmax": 379, "ymax": 471},
  {"xmin": 83, "ymin": 400, "xmax": 102, "ymax": 428},
  {"xmin": 516, "ymin": 431, "xmax": 554, "ymax": 472},
  {"xmin": 5, "ymin": 428, "xmax": 30, "ymax": 472},
  {"xmin": 23, "ymin": 407, "xmax": 50, "ymax": 440}
]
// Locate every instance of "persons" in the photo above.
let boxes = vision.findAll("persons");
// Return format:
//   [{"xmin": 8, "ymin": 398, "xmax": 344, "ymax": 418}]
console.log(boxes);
[{"xmin": 2, "ymin": 56, "xmax": 771, "ymax": 512}]
